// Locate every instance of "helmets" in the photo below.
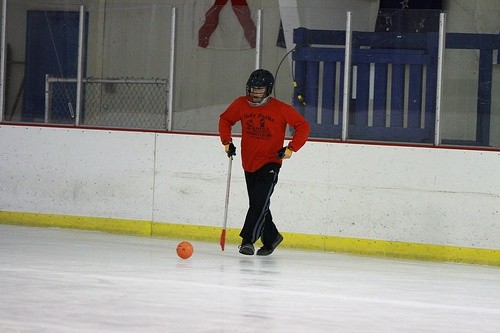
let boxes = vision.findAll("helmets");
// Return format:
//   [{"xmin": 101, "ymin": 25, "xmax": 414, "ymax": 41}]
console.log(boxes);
[{"xmin": 246, "ymin": 68, "xmax": 275, "ymax": 107}]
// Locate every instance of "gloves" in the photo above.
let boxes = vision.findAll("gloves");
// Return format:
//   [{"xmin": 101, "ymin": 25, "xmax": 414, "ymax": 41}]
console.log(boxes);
[
  {"xmin": 224, "ymin": 143, "xmax": 236, "ymax": 160},
  {"xmin": 276, "ymin": 147, "xmax": 292, "ymax": 160}
]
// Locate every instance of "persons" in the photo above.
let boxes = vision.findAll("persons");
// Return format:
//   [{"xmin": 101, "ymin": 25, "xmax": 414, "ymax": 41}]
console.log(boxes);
[{"xmin": 218, "ymin": 69, "xmax": 312, "ymax": 256}]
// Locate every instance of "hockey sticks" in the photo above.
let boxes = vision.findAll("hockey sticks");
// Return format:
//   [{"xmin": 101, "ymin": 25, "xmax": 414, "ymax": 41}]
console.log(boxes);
[{"xmin": 219, "ymin": 138, "xmax": 234, "ymax": 252}]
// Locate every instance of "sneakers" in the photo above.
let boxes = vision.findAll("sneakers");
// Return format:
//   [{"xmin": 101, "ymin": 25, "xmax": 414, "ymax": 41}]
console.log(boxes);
[
  {"xmin": 257, "ymin": 232, "xmax": 283, "ymax": 255},
  {"xmin": 237, "ymin": 238, "xmax": 255, "ymax": 255}
]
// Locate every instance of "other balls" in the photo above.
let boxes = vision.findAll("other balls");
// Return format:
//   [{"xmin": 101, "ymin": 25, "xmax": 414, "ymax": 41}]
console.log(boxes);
[{"xmin": 176, "ymin": 241, "xmax": 194, "ymax": 260}]
